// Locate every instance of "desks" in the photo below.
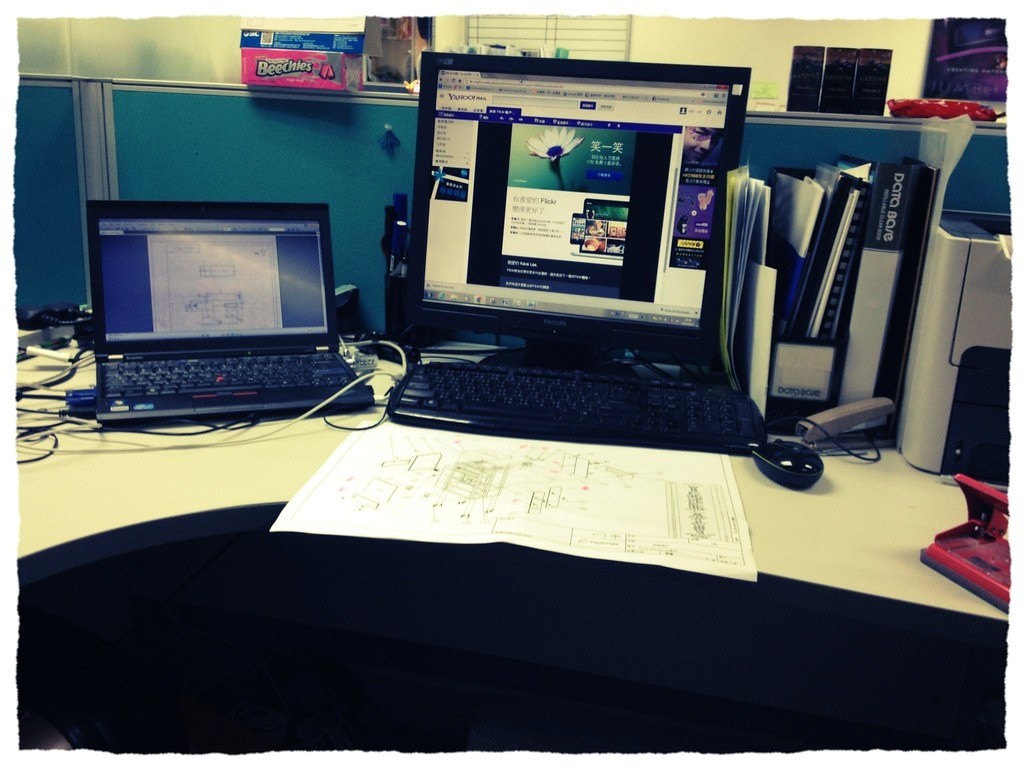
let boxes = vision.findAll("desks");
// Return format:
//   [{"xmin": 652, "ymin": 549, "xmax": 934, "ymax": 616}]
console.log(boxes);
[{"xmin": 18, "ymin": 352, "xmax": 1010, "ymax": 644}]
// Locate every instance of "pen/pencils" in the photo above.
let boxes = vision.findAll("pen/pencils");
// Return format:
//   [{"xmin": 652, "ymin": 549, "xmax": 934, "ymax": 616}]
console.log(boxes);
[{"xmin": 384, "ymin": 192, "xmax": 411, "ymax": 277}]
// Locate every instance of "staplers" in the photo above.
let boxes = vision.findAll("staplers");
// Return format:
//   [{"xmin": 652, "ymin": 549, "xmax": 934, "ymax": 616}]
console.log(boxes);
[{"xmin": 796, "ymin": 397, "xmax": 897, "ymax": 456}]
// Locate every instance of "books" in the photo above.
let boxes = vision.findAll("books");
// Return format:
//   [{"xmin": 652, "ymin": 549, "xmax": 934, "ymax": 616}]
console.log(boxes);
[{"xmin": 775, "ymin": 159, "xmax": 872, "ymax": 340}]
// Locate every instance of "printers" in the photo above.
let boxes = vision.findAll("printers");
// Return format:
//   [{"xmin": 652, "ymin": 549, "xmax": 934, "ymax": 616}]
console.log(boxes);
[{"xmin": 901, "ymin": 208, "xmax": 1013, "ymax": 486}]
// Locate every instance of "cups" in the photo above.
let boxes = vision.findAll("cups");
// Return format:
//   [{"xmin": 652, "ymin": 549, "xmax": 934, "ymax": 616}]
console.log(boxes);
[{"xmin": 787, "ymin": 44, "xmax": 892, "ymax": 116}]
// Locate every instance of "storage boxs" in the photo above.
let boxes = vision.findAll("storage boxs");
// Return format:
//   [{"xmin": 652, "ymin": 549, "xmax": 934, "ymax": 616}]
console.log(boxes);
[
  {"xmin": 240, "ymin": 30, "xmax": 366, "ymax": 55},
  {"xmin": 239, "ymin": 47, "xmax": 364, "ymax": 92}
]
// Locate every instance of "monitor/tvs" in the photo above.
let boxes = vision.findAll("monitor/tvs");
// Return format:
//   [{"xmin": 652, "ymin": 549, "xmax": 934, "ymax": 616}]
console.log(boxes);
[{"xmin": 404, "ymin": 50, "xmax": 752, "ymax": 376}]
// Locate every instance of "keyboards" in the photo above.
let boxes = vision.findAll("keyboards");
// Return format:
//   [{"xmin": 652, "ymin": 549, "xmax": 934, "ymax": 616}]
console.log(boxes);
[{"xmin": 387, "ymin": 364, "xmax": 768, "ymax": 458}]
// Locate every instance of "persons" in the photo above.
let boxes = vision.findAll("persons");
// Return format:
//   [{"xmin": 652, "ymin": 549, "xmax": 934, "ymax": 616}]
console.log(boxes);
[{"xmin": 685, "ymin": 127, "xmax": 721, "ymax": 165}]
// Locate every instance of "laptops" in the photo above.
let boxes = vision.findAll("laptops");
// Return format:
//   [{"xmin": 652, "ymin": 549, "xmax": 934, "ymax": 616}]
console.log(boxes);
[{"xmin": 85, "ymin": 199, "xmax": 375, "ymax": 428}]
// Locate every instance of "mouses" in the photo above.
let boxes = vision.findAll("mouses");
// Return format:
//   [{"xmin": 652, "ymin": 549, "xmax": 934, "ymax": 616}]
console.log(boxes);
[{"xmin": 751, "ymin": 439, "xmax": 824, "ymax": 491}]
